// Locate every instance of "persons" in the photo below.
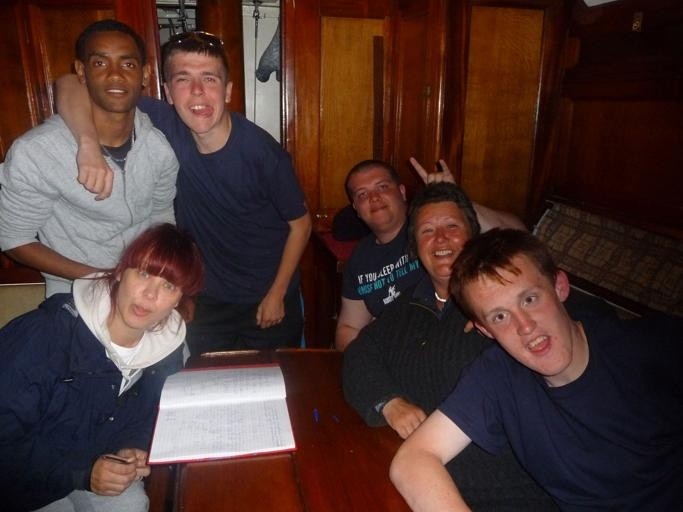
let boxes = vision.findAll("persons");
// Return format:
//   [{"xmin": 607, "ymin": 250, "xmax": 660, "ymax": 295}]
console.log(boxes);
[
  {"xmin": 0, "ymin": 18, "xmax": 180, "ymax": 299},
  {"xmin": 334, "ymin": 157, "xmax": 528, "ymax": 352},
  {"xmin": 341, "ymin": 181, "xmax": 499, "ymax": 436},
  {"xmin": 389, "ymin": 229, "xmax": 682, "ymax": 510},
  {"xmin": 0, "ymin": 223, "xmax": 206, "ymax": 511},
  {"xmin": 55, "ymin": 31, "xmax": 314, "ymax": 355}
]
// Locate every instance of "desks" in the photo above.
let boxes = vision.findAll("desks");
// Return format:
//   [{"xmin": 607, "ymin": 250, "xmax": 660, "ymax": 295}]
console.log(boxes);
[{"xmin": 142, "ymin": 343, "xmax": 414, "ymax": 511}]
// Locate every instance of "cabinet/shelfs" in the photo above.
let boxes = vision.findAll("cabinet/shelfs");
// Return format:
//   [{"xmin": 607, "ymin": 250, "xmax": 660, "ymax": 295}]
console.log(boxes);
[
  {"xmin": 279, "ymin": 1, "xmax": 554, "ymax": 348},
  {"xmin": 0, "ymin": 2, "xmax": 247, "ymax": 284}
]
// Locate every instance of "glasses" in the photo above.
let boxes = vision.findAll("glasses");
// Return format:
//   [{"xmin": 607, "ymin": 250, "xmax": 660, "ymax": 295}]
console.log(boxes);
[{"xmin": 167, "ymin": 30, "xmax": 225, "ymax": 50}]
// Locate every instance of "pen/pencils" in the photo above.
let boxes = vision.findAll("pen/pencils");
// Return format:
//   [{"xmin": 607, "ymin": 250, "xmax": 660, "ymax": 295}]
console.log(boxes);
[{"xmin": 102, "ymin": 452, "xmax": 130, "ymax": 464}]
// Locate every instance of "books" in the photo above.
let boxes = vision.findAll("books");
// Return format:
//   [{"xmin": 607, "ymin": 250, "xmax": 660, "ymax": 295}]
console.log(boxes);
[{"xmin": 147, "ymin": 361, "xmax": 296, "ymax": 465}]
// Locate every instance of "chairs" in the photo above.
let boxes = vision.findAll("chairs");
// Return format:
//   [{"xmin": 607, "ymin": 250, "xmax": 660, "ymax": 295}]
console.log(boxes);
[{"xmin": 0, "ymin": 283, "xmax": 47, "ymax": 328}]
